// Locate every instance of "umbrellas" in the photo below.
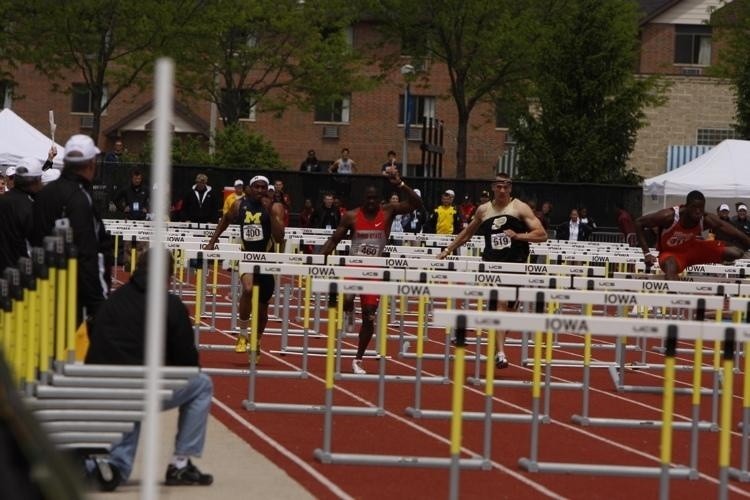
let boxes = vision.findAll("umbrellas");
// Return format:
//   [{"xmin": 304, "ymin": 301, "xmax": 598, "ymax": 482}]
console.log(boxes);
[{"xmin": 0, "ymin": 105, "xmax": 73, "ymax": 174}]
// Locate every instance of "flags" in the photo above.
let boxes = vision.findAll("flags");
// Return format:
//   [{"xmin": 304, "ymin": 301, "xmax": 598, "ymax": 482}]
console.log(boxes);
[{"xmin": 403, "ymin": 92, "xmax": 415, "ymax": 139}]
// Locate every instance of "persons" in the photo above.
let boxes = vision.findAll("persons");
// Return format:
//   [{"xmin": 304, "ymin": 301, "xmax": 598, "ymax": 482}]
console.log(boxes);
[
  {"xmin": 0, "ymin": 134, "xmax": 114, "ymax": 342},
  {"xmin": 100, "ymin": 140, "xmax": 430, "ymax": 233},
  {"xmin": 203, "ymin": 171, "xmax": 288, "ymax": 366},
  {"xmin": 317, "ymin": 166, "xmax": 422, "ymax": 377},
  {"xmin": 436, "ymin": 170, "xmax": 548, "ymax": 369},
  {"xmin": 426, "ymin": 189, "xmax": 750, "ymax": 295},
  {"xmin": 81, "ymin": 245, "xmax": 214, "ymax": 493}
]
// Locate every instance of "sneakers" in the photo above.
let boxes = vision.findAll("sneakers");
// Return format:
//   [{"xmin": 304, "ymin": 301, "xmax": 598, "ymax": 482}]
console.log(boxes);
[
  {"xmin": 166, "ymin": 459, "xmax": 212, "ymax": 485},
  {"xmin": 250, "ymin": 337, "xmax": 261, "ymax": 364},
  {"xmin": 352, "ymin": 359, "xmax": 366, "ymax": 374},
  {"xmin": 235, "ymin": 332, "xmax": 248, "ymax": 352},
  {"xmin": 346, "ymin": 308, "xmax": 355, "ymax": 332},
  {"xmin": 495, "ymin": 352, "xmax": 508, "ymax": 368}
]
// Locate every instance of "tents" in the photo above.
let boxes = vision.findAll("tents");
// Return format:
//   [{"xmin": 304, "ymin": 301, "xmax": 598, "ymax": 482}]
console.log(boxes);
[{"xmin": 642, "ymin": 140, "xmax": 750, "ymax": 231}]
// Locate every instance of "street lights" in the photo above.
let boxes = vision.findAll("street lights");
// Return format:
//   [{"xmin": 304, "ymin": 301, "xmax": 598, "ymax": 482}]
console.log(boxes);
[
  {"xmin": 208, "ymin": 50, "xmax": 240, "ymax": 164},
  {"xmin": 399, "ymin": 64, "xmax": 417, "ymax": 178}
]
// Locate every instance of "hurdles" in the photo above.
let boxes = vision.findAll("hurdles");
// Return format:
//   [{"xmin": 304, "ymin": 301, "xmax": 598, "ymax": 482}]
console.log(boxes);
[
  {"xmin": 0, "ymin": 226, "xmax": 202, "ymax": 455},
  {"xmin": 405, "ymin": 269, "xmax": 572, "ymax": 425},
  {"xmin": 517, "ymin": 286, "xmax": 725, "ymax": 482},
  {"xmin": 727, "ymin": 295, "xmax": 750, "ymax": 483},
  {"xmin": 571, "ymin": 276, "xmax": 740, "ymax": 432},
  {"xmin": 431, "ymin": 308, "xmax": 703, "ymax": 500},
  {"xmin": 102, "ymin": 219, "xmax": 750, "ymax": 398},
  {"xmin": 702, "ymin": 321, "xmax": 750, "ymax": 500},
  {"xmin": 310, "ymin": 278, "xmax": 517, "ymax": 471},
  {"xmin": 240, "ymin": 261, "xmax": 405, "ymax": 418}
]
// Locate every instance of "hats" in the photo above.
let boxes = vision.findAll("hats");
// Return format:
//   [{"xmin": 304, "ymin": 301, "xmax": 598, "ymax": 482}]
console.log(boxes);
[{"xmin": 6, "ymin": 135, "xmax": 101, "ymax": 177}]
